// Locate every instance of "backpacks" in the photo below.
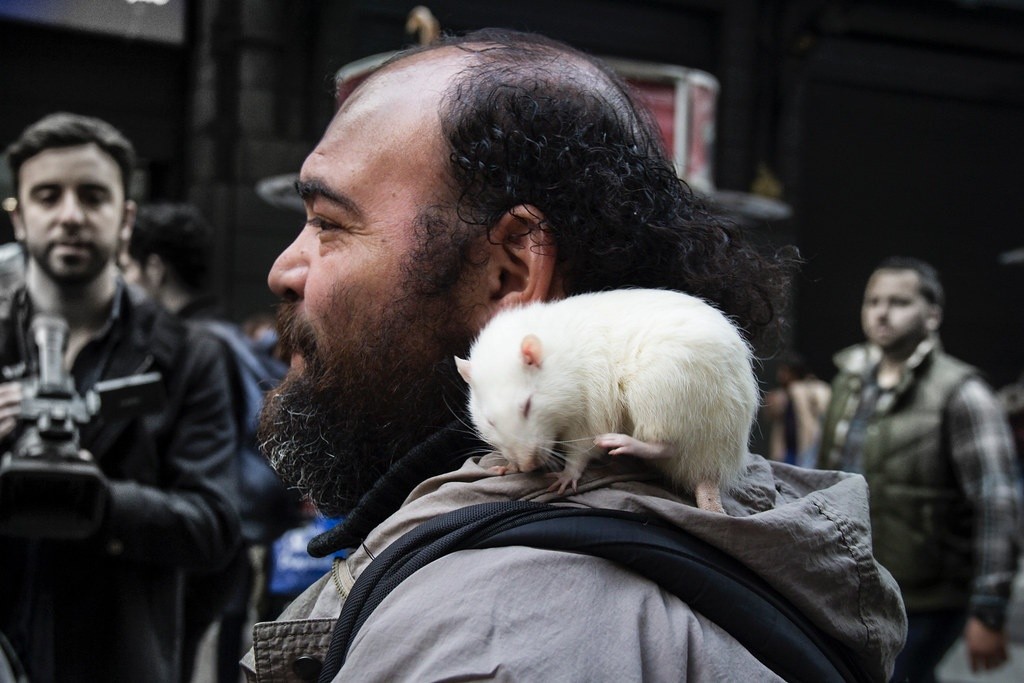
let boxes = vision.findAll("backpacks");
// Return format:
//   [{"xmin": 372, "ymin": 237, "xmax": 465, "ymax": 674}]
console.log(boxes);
[{"xmin": 198, "ymin": 319, "xmax": 296, "ymax": 547}]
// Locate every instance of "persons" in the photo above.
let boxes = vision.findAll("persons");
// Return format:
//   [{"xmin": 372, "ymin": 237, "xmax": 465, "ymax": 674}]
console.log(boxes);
[
  {"xmin": 239, "ymin": 31, "xmax": 908, "ymax": 683},
  {"xmin": 0, "ymin": 115, "xmax": 320, "ymax": 683},
  {"xmin": 760, "ymin": 255, "xmax": 1024, "ymax": 683}
]
